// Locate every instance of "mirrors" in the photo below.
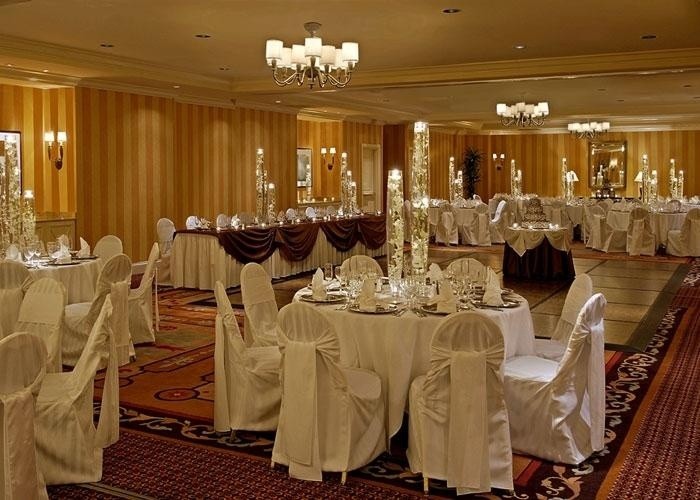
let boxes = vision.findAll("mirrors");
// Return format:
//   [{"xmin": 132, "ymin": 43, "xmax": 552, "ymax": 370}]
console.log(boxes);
[{"xmin": 588, "ymin": 141, "xmax": 627, "ymax": 190}]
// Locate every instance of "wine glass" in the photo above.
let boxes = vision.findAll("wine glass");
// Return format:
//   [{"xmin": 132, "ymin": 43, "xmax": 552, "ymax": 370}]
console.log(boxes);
[
  {"xmin": 301, "ymin": 258, "xmax": 519, "ymax": 314},
  {"xmin": 24, "ymin": 240, "xmax": 99, "ymax": 266}
]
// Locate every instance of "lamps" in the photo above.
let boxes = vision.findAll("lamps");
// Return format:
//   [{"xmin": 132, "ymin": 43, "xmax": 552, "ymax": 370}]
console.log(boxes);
[
  {"xmin": 634, "ymin": 171, "xmax": 643, "ymax": 200},
  {"xmin": 496, "ymin": 102, "xmax": 549, "ymax": 127},
  {"xmin": 321, "ymin": 148, "xmax": 336, "ymax": 170},
  {"xmin": 45, "ymin": 131, "xmax": 67, "ymax": 170},
  {"xmin": 568, "ymin": 121, "xmax": 611, "ymax": 139},
  {"xmin": 265, "ymin": 22, "xmax": 359, "ymax": 89},
  {"xmin": 568, "ymin": 172, "xmax": 579, "ymax": 181},
  {"xmin": 493, "ymin": 153, "xmax": 505, "ymax": 170}
]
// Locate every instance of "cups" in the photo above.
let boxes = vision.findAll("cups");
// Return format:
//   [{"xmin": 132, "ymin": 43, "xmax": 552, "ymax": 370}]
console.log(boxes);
[{"xmin": 195, "ymin": 212, "xmax": 381, "ymax": 233}]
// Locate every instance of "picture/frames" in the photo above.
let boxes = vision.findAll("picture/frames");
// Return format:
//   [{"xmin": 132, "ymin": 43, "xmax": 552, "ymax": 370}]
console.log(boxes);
[
  {"xmin": 0, "ymin": 131, "xmax": 23, "ymax": 195},
  {"xmin": 296, "ymin": 148, "xmax": 313, "ymax": 188}
]
